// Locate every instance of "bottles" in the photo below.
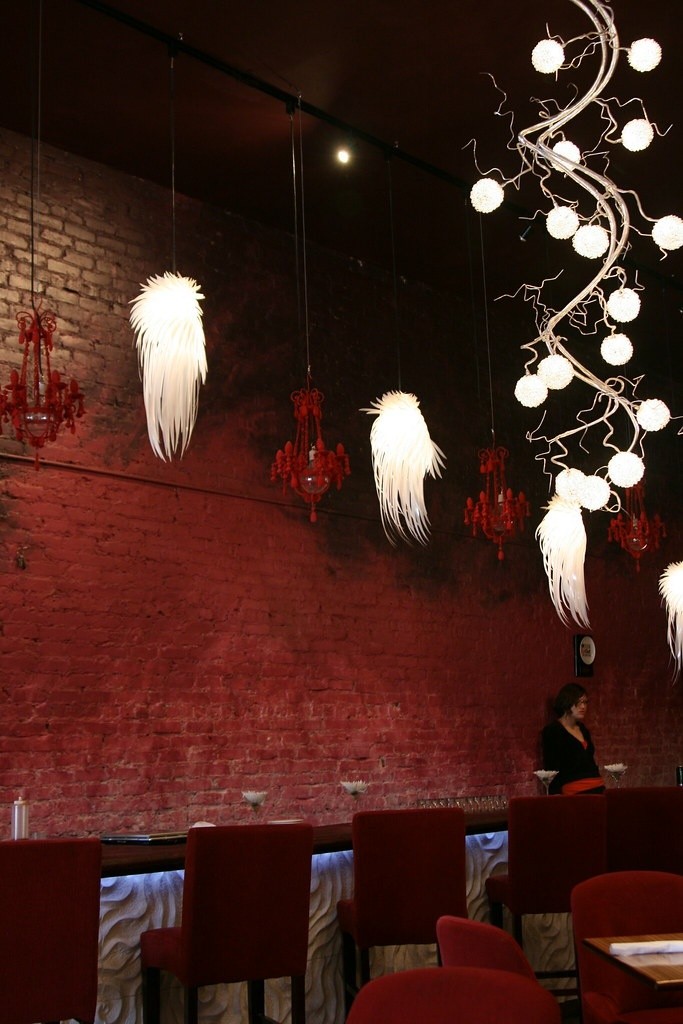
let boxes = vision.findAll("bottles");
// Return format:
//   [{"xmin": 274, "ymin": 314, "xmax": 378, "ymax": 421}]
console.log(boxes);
[{"xmin": 10, "ymin": 796, "xmax": 30, "ymax": 841}]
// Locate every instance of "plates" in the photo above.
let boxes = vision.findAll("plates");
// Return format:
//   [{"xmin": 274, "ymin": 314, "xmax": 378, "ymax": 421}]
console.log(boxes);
[{"xmin": 267, "ymin": 819, "xmax": 304, "ymax": 824}]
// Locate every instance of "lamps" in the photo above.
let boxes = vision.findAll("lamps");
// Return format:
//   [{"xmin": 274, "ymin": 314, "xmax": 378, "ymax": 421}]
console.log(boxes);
[{"xmin": 1, "ymin": 1, "xmax": 671, "ymax": 571}]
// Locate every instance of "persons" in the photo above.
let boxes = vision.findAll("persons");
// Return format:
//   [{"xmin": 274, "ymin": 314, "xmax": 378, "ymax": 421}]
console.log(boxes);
[{"xmin": 542, "ymin": 683, "xmax": 607, "ymax": 795}]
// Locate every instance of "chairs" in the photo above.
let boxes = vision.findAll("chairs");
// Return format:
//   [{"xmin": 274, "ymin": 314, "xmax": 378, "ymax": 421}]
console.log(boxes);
[{"xmin": 0, "ymin": 786, "xmax": 683, "ymax": 1022}]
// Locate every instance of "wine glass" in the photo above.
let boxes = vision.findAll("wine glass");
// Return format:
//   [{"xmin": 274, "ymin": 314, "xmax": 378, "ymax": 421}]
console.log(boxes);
[
  {"xmin": 604, "ymin": 764, "xmax": 628, "ymax": 789},
  {"xmin": 342, "ymin": 783, "xmax": 368, "ymax": 812},
  {"xmin": 533, "ymin": 771, "xmax": 558, "ymax": 795},
  {"xmin": 242, "ymin": 790, "xmax": 268, "ymax": 822}
]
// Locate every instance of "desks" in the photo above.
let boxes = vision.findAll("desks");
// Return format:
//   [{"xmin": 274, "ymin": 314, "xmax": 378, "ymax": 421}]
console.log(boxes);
[
  {"xmin": 94, "ymin": 810, "xmax": 580, "ymax": 1023},
  {"xmin": 583, "ymin": 931, "xmax": 682, "ymax": 989}
]
